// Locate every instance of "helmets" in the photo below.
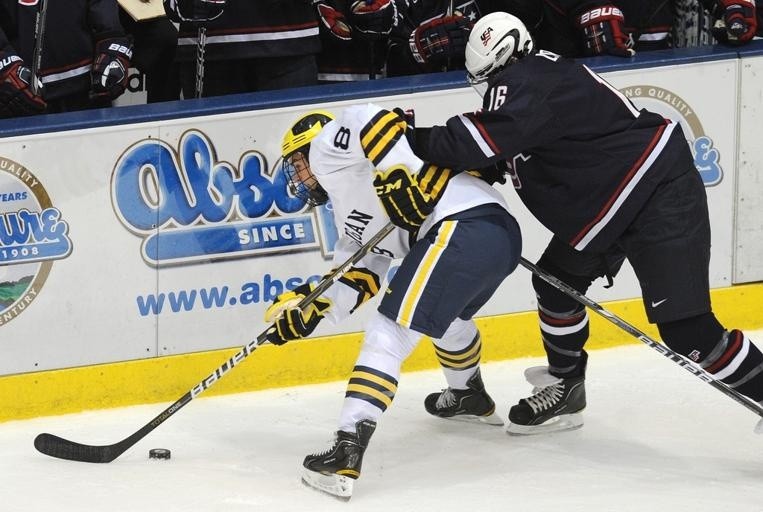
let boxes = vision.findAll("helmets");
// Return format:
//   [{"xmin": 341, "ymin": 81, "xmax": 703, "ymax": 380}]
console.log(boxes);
[
  {"xmin": 463, "ymin": 11, "xmax": 534, "ymax": 99},
  {"xmin": 281, "ymin": 109, "xmax": 337, "ymax": 209}
]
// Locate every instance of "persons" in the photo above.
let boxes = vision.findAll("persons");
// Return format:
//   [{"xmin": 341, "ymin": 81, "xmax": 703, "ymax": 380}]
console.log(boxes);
[
  {"xmin": 401, "ymin": 11, "xmax": 763, "ymax": 426},
  {"xmin": 264, "ymin": 110, "xmax": 523, "ymax": 477}
]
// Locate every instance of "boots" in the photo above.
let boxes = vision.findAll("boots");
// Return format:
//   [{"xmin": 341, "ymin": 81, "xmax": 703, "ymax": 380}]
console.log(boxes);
[
  {"xmin": 509, "ymin": 350, "xmax": 587, "ymax": 425},
  {"xmin": 303, "ymin": 419, "xmax": 376, "ymax": 479},
  {"xmin": 424, "ymin": 366, "xmax": 494, "ymax": 418}
]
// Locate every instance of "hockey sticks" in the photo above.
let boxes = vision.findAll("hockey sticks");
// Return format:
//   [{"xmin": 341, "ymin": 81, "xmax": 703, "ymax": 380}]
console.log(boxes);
[{"xmin": 33, "ymin": 222, "xmax": 396, "ymax": 464}]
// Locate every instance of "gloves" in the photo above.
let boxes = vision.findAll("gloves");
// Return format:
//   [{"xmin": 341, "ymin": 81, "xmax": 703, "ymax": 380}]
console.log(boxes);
[
  {"xmin": 263, "ymin": 282, "xmax": 331, "ymax": 346},
  {"xmin": 89, "ymin": 32, "xmax": 137, "ymax": 102},
  {"xmin": 710, "ymin": 0, "xmax": 759, "ymax": 47},
  {"xmin": 403, "ymin": 13, "xmax": 466, "ymax": 68},
  {"xmin": 372, "ymin": 164, "xmax": 435, "ymax": 232},
  {"xmin": 1, "ymin": 56, "xmax": 49, "ymax": 115},
  {"xmin": 575, "ymin": 4, "xmax": 637, "ymax": 58}
]
